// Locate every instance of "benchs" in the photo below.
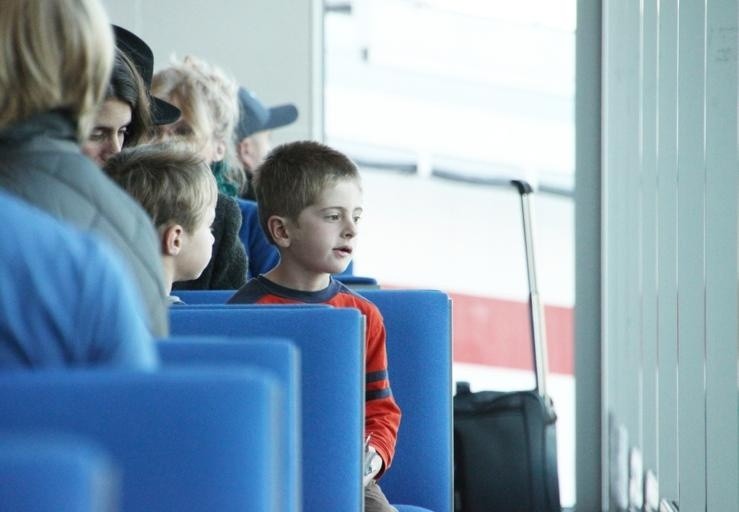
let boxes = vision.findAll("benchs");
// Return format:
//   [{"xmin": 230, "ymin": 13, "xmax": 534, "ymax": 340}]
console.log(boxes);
[{"xmin": 0, "ymin": 276, "xmax": 455, "ymax": 512}]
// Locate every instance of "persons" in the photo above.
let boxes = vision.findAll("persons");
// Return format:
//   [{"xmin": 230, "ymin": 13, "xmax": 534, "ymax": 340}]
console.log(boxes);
[{"xmin": 221, "ymin": 140, "xmax": 403, "ymax": 511}]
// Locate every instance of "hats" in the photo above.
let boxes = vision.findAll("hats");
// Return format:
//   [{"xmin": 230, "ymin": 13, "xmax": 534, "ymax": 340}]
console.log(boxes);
[
  {"xmin": 234, "ymin": 86, "xmax": 298, "ymax": 142},
  {"xmin": 110, "ymin": 22, "xmax": 181, "ymax": 129}
]
